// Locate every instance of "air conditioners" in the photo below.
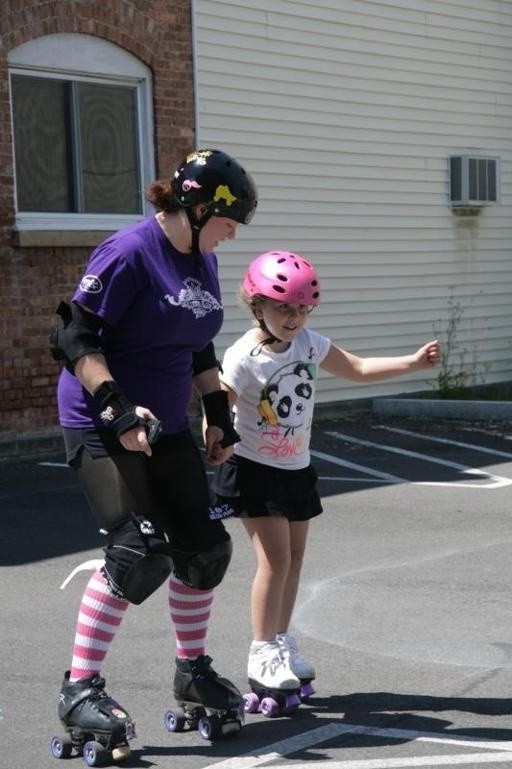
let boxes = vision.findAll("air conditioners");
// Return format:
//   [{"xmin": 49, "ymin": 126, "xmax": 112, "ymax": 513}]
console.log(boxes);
[{"xmin": 449, "ymin": 154, "xmax": 501, "ymax": 206}]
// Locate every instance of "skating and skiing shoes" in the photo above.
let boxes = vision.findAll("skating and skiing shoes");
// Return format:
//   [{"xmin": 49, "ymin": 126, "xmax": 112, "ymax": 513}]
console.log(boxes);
[
  {"xmin": 242, "ymin": 633, "xmax": 316, "ymax": 717},
  {"xmin": 163, "ymin": 655, "xmax": 242, "ymax": 739},
  {"xmin": 50, "ymin": 669, "xmax": 137, "ymax": 767}
]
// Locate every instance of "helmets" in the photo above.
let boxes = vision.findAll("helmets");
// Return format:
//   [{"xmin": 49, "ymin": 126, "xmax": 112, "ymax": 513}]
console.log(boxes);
[
  {"xmin": 170, "ymin": 149, "xmax": 257, "ymax": 224},
  {"xmin": 243, "ymin": 251, "xmax": 321, "ymax": 307}
]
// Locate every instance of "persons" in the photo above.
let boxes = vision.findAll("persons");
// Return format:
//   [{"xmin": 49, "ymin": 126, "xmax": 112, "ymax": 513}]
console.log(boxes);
[
  {"xmin": 197, "ymin": 248, "xmax": 442, "ymax": 690},
  {"xmin": 51, "ymin": 148, "xmax": 257, "ymax": 724}
]
